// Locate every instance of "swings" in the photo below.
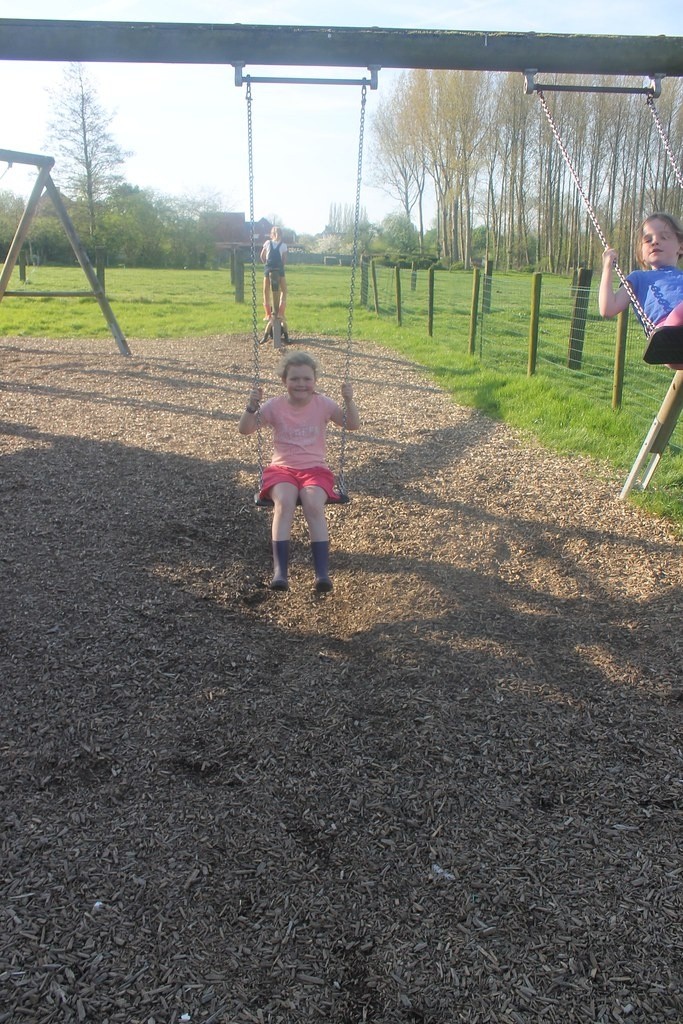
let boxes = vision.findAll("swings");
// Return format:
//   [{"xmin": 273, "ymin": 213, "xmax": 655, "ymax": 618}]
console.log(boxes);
[
  {"xmin": 526, "ymin": 82, "xmax": 683, "ymax": 367},
  {"xmin": 243, "ymin": 77, "xmax": 364, "ymax": 510}
]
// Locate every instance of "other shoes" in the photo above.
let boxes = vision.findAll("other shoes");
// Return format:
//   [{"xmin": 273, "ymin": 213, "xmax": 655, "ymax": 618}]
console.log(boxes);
[
  {"xmin": 285, "ymin": 338, "xmax": 290, "ymax": 343},
  {"xmin": 259, "ymin": 338, "xmax": 267, "ymax": 344},
  {"xmin": 262, "ymin": 314, "xmax": 271, "ymax": 321},
  {"xmin": 315, "ymin": 576, "xmax": 333, "ymax": 593},
  {"xmin": 276, "ymin": 313, "xmax": 286, "ymax": 320},
  {"xmin": 270, "ymin": 577, "xmax": 288, "ymax": 591}
]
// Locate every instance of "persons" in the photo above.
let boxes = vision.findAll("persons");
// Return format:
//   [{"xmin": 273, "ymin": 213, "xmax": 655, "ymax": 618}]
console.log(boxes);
[
  {"xmin": 260, "ymin": 226, "xmax": 288, "ymax": 321},
  {"xmin": 598, "ymin": 213, "xmax": 683, "ymax": 370},
  {"xmin": 239, "ymin": 353, "xmax": 360, "ymax": 592}
]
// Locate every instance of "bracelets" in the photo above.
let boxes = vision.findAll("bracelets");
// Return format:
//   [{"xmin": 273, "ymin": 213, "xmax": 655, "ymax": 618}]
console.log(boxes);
[{"xmin": 245, "ymin": 405, "xmax": 257, "ymax": 414}]
